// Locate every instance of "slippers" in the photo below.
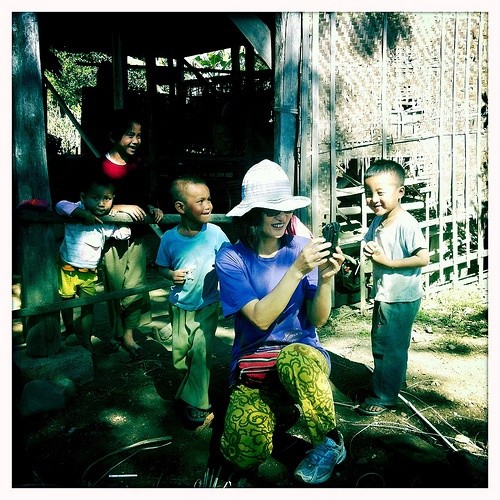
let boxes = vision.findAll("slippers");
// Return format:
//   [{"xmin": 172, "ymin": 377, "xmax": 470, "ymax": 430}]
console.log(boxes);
[
  {"xmin": 357, "ymin": 398, "xmax": 394, "ymax": 414},
  {"xmin": 103, "ymin": 335, "xmax": 120, "ymax": 353},
  {"xmin": 121, "ymin": 337, "xmax": 146, "ymax": 359},
  {"xmin": 338, "ymin": 375, "xmax": 369, "ymax": 391}
]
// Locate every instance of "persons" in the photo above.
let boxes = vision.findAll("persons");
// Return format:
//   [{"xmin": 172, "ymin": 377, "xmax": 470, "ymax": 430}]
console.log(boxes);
[
  {"xmin": 215, "ymin": 159, "xmax": 347, "ymax": 484},
  {"xmin": 358, "ymin": 160, "xmax": 430, "ymax": 415},
  {"xmin": 55, "ymin": 175, "xmax": 131, "ymax": 354},
  {"xmin": 88, "ymin": 108, "xmax": 163, "ymax": 360},
  {"xmin": 154, "ymin": 177, "xmax": 230, "ymax": 425}
]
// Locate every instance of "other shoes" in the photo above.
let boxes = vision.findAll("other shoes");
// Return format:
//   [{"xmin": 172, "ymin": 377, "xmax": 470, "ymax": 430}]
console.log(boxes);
[
  {"xmin": 208, "ymin": 364, "xmax": 224, "ymax": 396},
  {"xmin": 184, "ymin": 404, "xmax": 205, "ymax": 421},
  {"xmin": 81, "ymin": 337, "xmax": 98, "ymax": 355},
  {"xmin": 64, "ymin": 328, "xmax": 80, "ymax": 346}
]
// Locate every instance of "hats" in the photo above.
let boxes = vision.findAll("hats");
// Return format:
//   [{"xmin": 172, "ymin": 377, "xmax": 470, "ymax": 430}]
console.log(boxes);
[{"xmin": 225, "ymin": 159, "xmax": 311, "ymax": 217}]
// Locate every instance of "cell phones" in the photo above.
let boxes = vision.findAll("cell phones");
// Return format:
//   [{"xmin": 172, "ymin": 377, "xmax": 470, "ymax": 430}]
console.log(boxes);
[{"xmin": 322, "ymin": 222, "xmax": 340, "ymax": 259}]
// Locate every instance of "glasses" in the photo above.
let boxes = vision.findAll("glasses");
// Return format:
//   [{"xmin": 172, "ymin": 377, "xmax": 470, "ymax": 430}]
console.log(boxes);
[{"xmin": 260, "ymin": 207, "xmax": 296, "ymax": 217}]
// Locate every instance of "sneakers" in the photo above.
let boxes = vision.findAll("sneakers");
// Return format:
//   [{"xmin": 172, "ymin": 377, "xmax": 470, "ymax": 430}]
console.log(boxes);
[
  {"xmin": 273, "ymin": 402, "xmax": 301, "ymax": 434},
  {"xmin": 295, "ymin": 430, "xmax": 349, "ymax": 483}
]
